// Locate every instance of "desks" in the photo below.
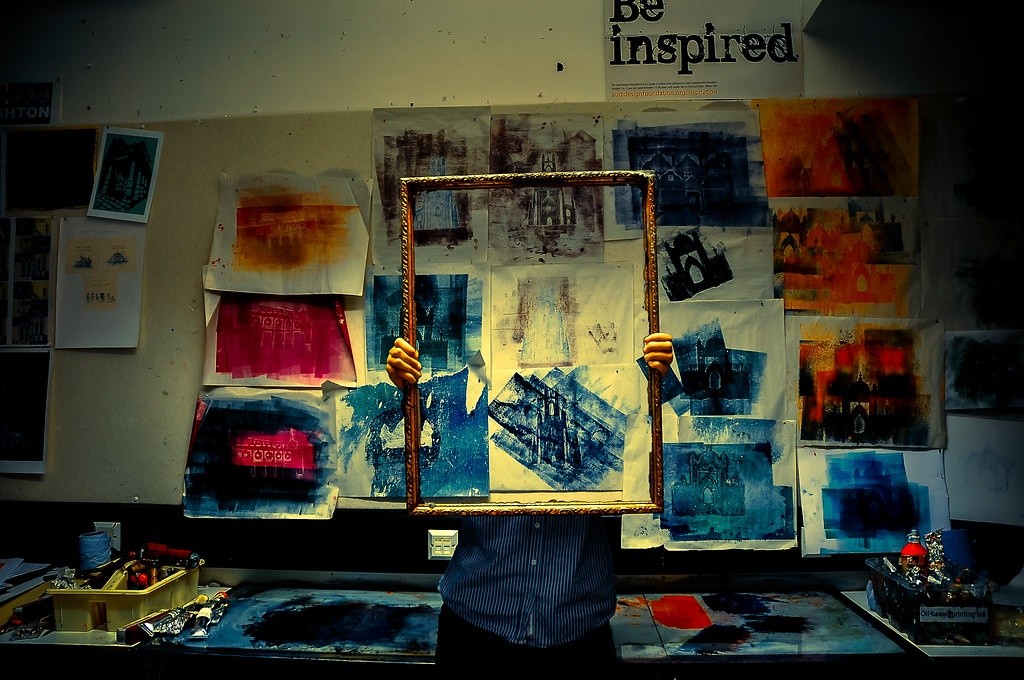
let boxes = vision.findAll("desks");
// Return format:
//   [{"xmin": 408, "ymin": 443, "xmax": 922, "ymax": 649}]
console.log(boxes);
[{"xmin": 0, "ymin": 577, "xmax": 1024, "ymax": 680}]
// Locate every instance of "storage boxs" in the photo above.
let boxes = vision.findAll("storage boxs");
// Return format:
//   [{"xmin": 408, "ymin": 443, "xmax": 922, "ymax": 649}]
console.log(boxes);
[
  {"xmin": 865, "ymin": 552, "xmax": 1001, "ymax": 646},
  {"xmin": 45, "ymin": 558, "xmax": 206, "ymax": 633}
]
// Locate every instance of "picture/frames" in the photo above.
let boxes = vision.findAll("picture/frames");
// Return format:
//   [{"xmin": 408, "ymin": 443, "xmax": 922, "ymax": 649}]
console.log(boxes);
[{"xmin": 398, "ymin": 170, "xmax": 668, "ymax": 517}]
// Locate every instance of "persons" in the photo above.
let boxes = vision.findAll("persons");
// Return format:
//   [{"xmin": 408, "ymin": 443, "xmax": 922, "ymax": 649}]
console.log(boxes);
[{"xmin": 385, "ymin": 332, "xmax": 674, "ymax": 680}]
